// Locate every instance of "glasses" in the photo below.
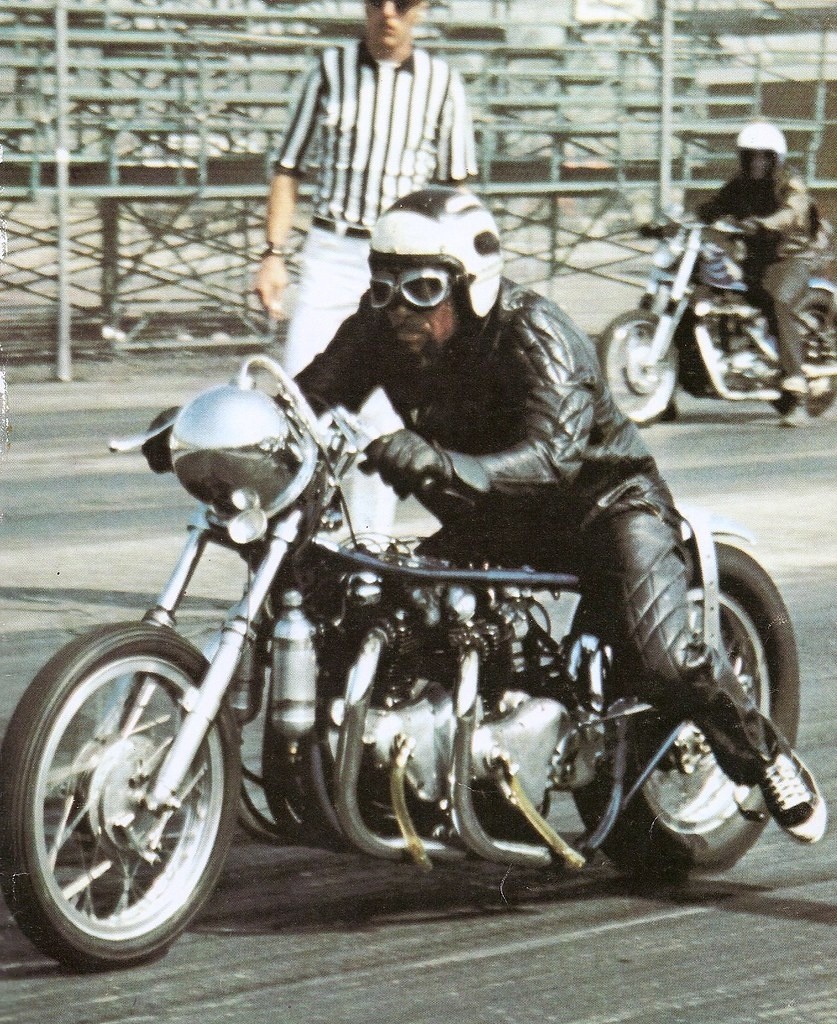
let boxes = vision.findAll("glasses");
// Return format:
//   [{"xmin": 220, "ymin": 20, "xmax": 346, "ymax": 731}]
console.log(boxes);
[
  {"xmin": 371, "ymin": 0, "xmax": 418, "ymax": 12},
  {"xmin": 368, "ymin": 267, "xmax": 460, "ymax": 312}
]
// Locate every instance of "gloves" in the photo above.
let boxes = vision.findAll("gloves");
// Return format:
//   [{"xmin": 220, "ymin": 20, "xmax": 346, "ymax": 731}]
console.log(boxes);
[
  {"xmin": 355, "ymin": 425, "xmax": 454, "ymax": 502},
  {"xmin": 140, "ymin": 405, "xmax": 184, "ymax": 476}
]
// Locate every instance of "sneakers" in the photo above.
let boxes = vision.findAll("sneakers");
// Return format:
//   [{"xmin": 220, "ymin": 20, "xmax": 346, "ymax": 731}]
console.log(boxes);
[{"xmin": 757, "ymin": 747, "xmax": 827, "ymax": 844}]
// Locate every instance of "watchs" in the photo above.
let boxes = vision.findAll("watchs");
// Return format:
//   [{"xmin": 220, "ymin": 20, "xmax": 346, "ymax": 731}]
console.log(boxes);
[{"xmin": 257, "ymin": 242, "xmax": 289, "ymax": 257}]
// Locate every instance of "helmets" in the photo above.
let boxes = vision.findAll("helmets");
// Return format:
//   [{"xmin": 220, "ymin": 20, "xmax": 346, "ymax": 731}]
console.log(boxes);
[
  {"xmin": 734, "ymin": 122, "xmax": 790, "ymax": 169},
  {"xmin": 369, "ymin": 182, "xmax": 503, "ymax": 318}
]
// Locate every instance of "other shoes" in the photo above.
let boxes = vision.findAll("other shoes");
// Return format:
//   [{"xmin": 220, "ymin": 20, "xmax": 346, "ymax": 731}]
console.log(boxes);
[{"xmin": 782, "ymin": 406, "xmax": 813, "ymax": 428}]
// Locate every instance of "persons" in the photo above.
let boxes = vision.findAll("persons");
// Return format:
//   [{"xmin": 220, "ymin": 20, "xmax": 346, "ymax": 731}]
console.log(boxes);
[
  {"xmin": 249, "ymin": 0, "xmax": 484, "ymax": 552},
  {"xmin": 642, "ymin": 119, "xmax": 821, "ymax": 394},
  {"xmin": 143, "ymin": 192, "xmax": 830, "ymax": 847}
]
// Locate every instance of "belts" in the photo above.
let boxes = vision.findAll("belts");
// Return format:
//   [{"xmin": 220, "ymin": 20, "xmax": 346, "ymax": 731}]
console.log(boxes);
[{"xmin": 311, "ymin": 215, "xmax": 372, "ymax": 239}]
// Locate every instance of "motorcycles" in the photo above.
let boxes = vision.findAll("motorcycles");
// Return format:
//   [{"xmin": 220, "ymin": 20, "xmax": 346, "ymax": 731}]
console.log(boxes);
[
  {"xmin": 595, "ymin": 222, "xmax": 836, "ymax": 429},
  {"xmin": 1, "ymin": 352, "xmax": 800, "ymax": 974}
]
